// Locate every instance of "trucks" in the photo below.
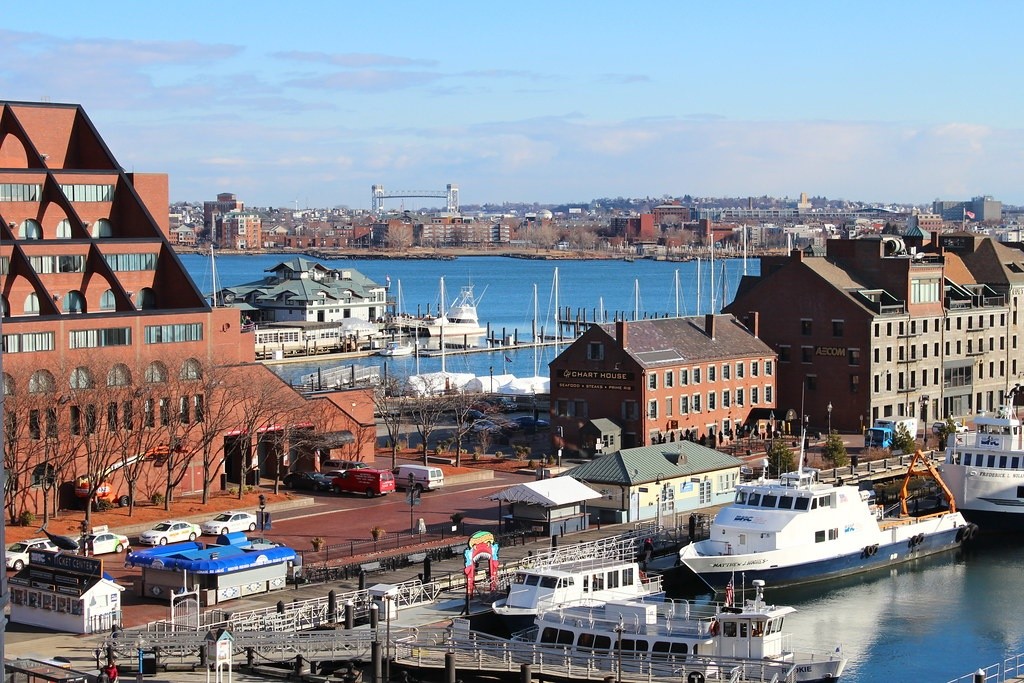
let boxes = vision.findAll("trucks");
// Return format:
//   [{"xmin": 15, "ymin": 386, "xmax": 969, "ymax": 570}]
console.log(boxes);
[
  {"xmin": 864, "ymin": 416, "xmax": 918, "ymax": 449},
  {"xmin": 932, "ymin": 421, "xmax": 950, "ymax": 434}
]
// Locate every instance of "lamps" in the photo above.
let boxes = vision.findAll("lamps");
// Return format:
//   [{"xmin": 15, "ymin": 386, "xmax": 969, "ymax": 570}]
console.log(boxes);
[
  {"xmin": 127, "ymin": 291, "xmax": 136, "ymax": 298},
  {"xmin": 53, "ymin": 294, "xmax": 62, "ymax": 302},
  {"xmin": 9, "ymin": 222, "xmax": 19, "ymax": 229},
  {"xmin": 83, "ymin": 221, "xmax": 93, "ymax": 230},
  {"xmin": 41, "ymin": 154, "xmax": 50, "ymax": 161}
]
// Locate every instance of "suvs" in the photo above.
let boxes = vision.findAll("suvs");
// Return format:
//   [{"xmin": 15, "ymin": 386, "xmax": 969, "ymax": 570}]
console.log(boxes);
[{"xmin": 5, "ymin": 538, "xmax": 60, "ymax": 571}]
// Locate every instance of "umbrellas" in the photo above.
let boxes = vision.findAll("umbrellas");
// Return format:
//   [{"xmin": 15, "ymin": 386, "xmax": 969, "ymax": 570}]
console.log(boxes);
[{"xmin": 658, "ymin": 424, "xmax": 772, "ymax": 449}]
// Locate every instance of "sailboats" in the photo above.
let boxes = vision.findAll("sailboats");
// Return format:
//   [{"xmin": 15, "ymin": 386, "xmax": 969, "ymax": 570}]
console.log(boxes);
[{"xmin": 377, "ymin": 266, "xmax": 563, "ymax": 395}]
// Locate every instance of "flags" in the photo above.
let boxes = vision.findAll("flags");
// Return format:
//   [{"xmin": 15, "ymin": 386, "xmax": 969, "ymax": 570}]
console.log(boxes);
[
  {"xmin": 504, "ymin": 354, "xmax": 512, "ymax": 363},
  {"xmin": 963, "ymin": 208, "xmax": 975, "ymax": 219},
  {"xmin": 723, "ymin": 575, "xmax": 733, "ymax": 608}
]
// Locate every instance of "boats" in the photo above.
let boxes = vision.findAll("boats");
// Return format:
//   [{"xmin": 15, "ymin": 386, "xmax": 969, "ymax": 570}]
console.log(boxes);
[
  {"xmin": 490, "ymin": 550, "xmax": 666, "ymax": 625},
  {"xmin": 677, "ymin": 428, "xmax": 980, "ymax": 593},
  {"xmin": 505, "ymin": 580, "xmax": 849, "ymax": 683},
  {"xmin": 936, "ymin": 386, "xmax": 1024, "ymax": 517}
]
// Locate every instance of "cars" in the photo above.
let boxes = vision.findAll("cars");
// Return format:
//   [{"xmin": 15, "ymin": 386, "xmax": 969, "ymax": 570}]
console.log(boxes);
[
  {"xmin": 452, "ymin": 395, "xmax": 552, "ymax": 433},
  {"xmin": 202, "ymin": 511, "xmax": 257, "ymax": 536},
  {"xmin": 953, "ymin": 422, "xmax": 969, "ymax": 432},
  {"xmin": 139, "ymin": 520, "xmax": 202, "ymax": 546},
  {"xmin": 74, "ymin": 532, "xmax": 130, "ymax": 556},
  {"xmin": 283, "ymin": 472, "xmax": 333, "ymax": 492}
]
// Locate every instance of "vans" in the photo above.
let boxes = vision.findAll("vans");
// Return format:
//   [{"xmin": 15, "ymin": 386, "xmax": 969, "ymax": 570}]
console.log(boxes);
[
  {"xmin": 392, "ymin": 464, "xmax": 445, "ymax": 492},
  {"xmin": 332, "ymin": 469, "xmax": 396, "ymax": 498}
]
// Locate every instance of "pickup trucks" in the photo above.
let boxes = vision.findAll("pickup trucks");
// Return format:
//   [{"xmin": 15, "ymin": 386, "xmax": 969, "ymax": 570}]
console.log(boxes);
[{"xmin": 321, "ymin": 460, "xmax": 369, "ymax": 475}]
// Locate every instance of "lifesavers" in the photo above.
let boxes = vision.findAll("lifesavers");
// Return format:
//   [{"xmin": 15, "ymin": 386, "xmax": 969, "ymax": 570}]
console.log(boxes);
[
  {"xmin": 872, "ymin": 545, "xmax": 879, "ymax": 555},
  {"xmin": 969, "ymin": 524, "xmax": 979, "ymax": 540},
  {"xmin": 866, "ymin": 546, "xmax": 873, "ymax": 556},
  {"xmin": 919, "ymin": 534, "xmax": 925, "ymax": 545},
  {"xmin": 710, "ymin": 621, "xmax": 720, "ymax": 636},
  {"xmin": 912, "ymin": 536, "xmax": 920, "ymax": 545},
  {"xmin": 959, "ymin": 527, "xmax": 970, "ymax": 541}
]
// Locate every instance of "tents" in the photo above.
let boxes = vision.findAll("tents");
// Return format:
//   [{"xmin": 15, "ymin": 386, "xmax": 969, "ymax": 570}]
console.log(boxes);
[{"xmin": 403, "ymin": 370, "xmax": 550, "ymax": 397}]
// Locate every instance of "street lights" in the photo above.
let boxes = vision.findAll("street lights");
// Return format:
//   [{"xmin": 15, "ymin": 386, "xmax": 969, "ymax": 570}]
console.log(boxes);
[
  {"xmin": 258, "ymin": 494, "xmax": 265, "ymax": 544},
  {"xmin": 385, "ymin": 595, "xmax": 396, "ymax": 683},
  {"xmin": 407, "ymin": 473, "xmax": 416, "ymax": 537},
  {"xmin": 827, "ymin": 401, "xmax": 833, "ymax": 441}
]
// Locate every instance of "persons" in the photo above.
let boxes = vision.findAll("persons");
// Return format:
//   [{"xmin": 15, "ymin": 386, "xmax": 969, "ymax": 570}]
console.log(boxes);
[
  {"xmin": 123, "ymin": 545, "xmax": 135, "ymax": 569},
  {"xmin": 97, "ymin": 661, "xmax": 117, "ymax": 683},
  {"xmin": 644, "ymin": 537, "xmax": 653, "ymax": 564},
  {"xmin": 528, "ymin": 550, "xmax": 532, "ymax": 563}
]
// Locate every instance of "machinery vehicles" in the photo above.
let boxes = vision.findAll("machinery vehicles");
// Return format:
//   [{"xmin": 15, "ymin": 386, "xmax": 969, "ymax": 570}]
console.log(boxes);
[
  {"xmin": 74, "ymin": 437, "xmax": 187, "ymax": 511},
  {"xmin": 870, "ymin": 447, "xmax": 956, "ymax": 530}
]
